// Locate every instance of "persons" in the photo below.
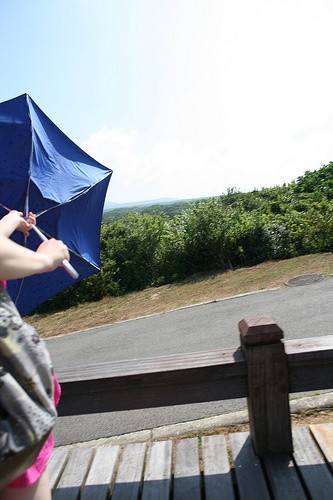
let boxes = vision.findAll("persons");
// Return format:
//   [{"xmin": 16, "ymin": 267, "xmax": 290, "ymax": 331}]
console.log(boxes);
[{"xmin": 0, "ymin": 211, "xmax": 71, "ymax": 500}]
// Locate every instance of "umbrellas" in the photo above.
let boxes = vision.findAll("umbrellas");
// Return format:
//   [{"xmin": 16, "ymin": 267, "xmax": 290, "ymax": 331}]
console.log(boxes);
[{"xmin": 0, "ymin": 91, "xmax": 114, "ymax": 321}]
